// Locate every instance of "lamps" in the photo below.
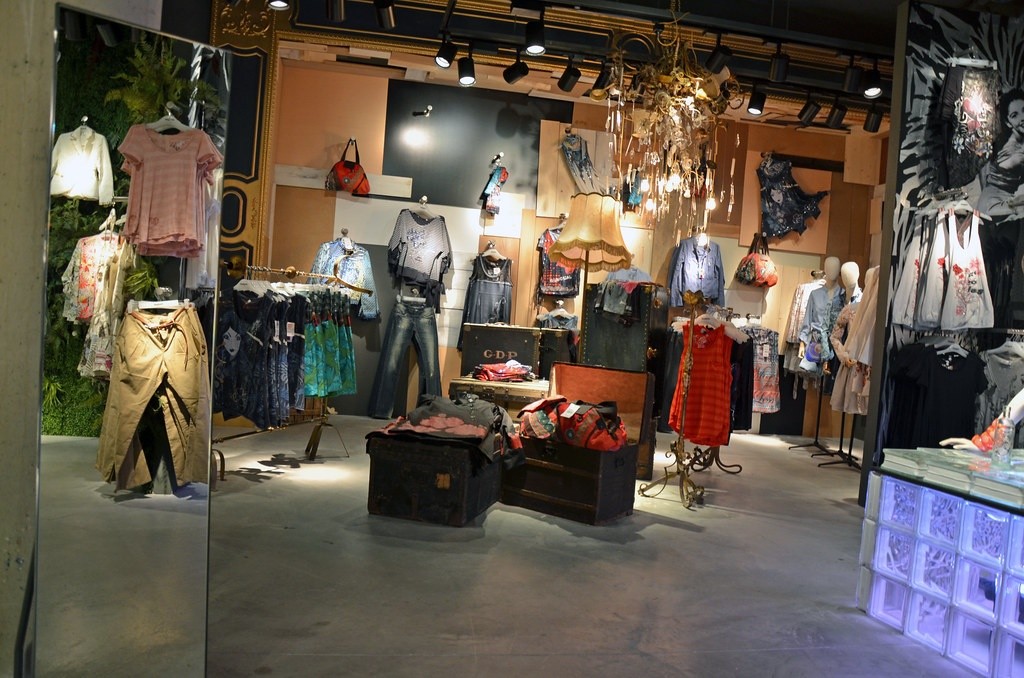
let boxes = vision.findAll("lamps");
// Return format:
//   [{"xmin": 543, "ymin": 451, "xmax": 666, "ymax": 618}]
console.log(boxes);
[
  {"xmin": 434, "ymin": 0, "xmax": 887, "ymax": 134},
  {"xmin": 547, "ymin": 192, "xmax": 629, "ymax": 366}
]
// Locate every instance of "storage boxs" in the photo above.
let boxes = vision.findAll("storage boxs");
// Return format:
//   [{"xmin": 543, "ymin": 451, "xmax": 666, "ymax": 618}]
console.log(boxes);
[
  {"xmin": 508, "ymin": 359, "xmax": 656, "ymax": 525},
  {"xmin": 366, "ymin": 427, "xmax": 509, "ymax": 529},
  {"xmin": 462, "ymin": 322, "xmax": 570, "ymax": 379}
]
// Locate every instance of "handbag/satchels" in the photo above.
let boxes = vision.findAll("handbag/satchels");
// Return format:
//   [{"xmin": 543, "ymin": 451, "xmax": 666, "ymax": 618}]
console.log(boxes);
[
  {"xmin": 734, "ymin": 233, "xmax": 778, "ymax": 287},
  {"xmin": 556, "ymin": 400, "xmax": 627, "ymax": 452},
  {"xmin": 325, "ymin": 139, "xmax": 371, "ymax": 194}
]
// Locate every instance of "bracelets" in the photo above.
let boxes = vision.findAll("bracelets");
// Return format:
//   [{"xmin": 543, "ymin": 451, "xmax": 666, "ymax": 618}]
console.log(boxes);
[{"xmin": 972, "ymin": 418, "xmax": 1008, "ymax": 453}]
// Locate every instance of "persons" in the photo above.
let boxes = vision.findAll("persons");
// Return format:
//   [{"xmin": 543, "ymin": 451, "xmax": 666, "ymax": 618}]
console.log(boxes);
[
  {"xmin": 939, "ymin": 387, "xmax": 1024, "ymax": 452},
  {"xmin": 829, "ymin": 267, "xmax": 876, "ymax": 417},
  {"xmin": 798, "ymin": 255, "xmax": 864, "ymax": 376},
  {"xmin": 997, "ymin": 88, "xmax": 1024, "ymax": 171}
]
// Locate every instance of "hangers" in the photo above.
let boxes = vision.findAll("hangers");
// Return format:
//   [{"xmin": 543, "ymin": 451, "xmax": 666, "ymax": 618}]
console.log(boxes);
[
  {"xmin": 143, "ymin": 99, "xmax": 196, "ymax": 133},
  {"xmin": 99, "ymin": 201, "xmax": 117, "ymax": 231},
  {"xmin": 233, "ymin": 266, "xmax": 351, "ymax": 302},
  {"xmin": 128, "ymin": 287, "xmax": 195, "ymax": 314},
  {"xmin": 694, "ymin": 225, "xmax": 709, "ymax": 246},
  {"xmin": 911, "ymin": 327, "xmax": 972, "ymax": 359},
  {"xmin": 907, "ymin": 187, "xmax": 994, "ymax": 225},
  {"xmin": 671, "ymin": 304, "xmax": 750, "ymax": 344},
  {"xmin": 946, "ymin": 45, "xmax": 998, "ymax": 70},
  {"xmin": 471, "ymin": 239, "xmax": 514, "ymax": 265},
  {"xmin": 985, "ymin": 328, "xmax": 1024, "ymax": 361},
  {"xmin": 397, "ymin": 288, "xmax": 429, "ymax": 304},
  {"xmin": 555, "ymin": 127, "xmax": 588, "ymax": 151},
  {"xmin": 79, "ymin": 117, "xmax": 89, "ymax": 129},
  {"xmin": 409, "ymin": 196, "xmax": 440, "ymax": 219}
]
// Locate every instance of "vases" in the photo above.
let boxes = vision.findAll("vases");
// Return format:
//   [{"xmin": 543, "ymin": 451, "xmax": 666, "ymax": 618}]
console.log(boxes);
[{"xmin": 991, "ymin": 406, "xmax": 1015, "ymax": 471}]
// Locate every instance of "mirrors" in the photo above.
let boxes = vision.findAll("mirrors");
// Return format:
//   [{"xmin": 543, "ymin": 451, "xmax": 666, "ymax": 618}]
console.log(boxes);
[{"xmin": 21, "ymin": 3, "xmax": 232, "ymax": 678}]
